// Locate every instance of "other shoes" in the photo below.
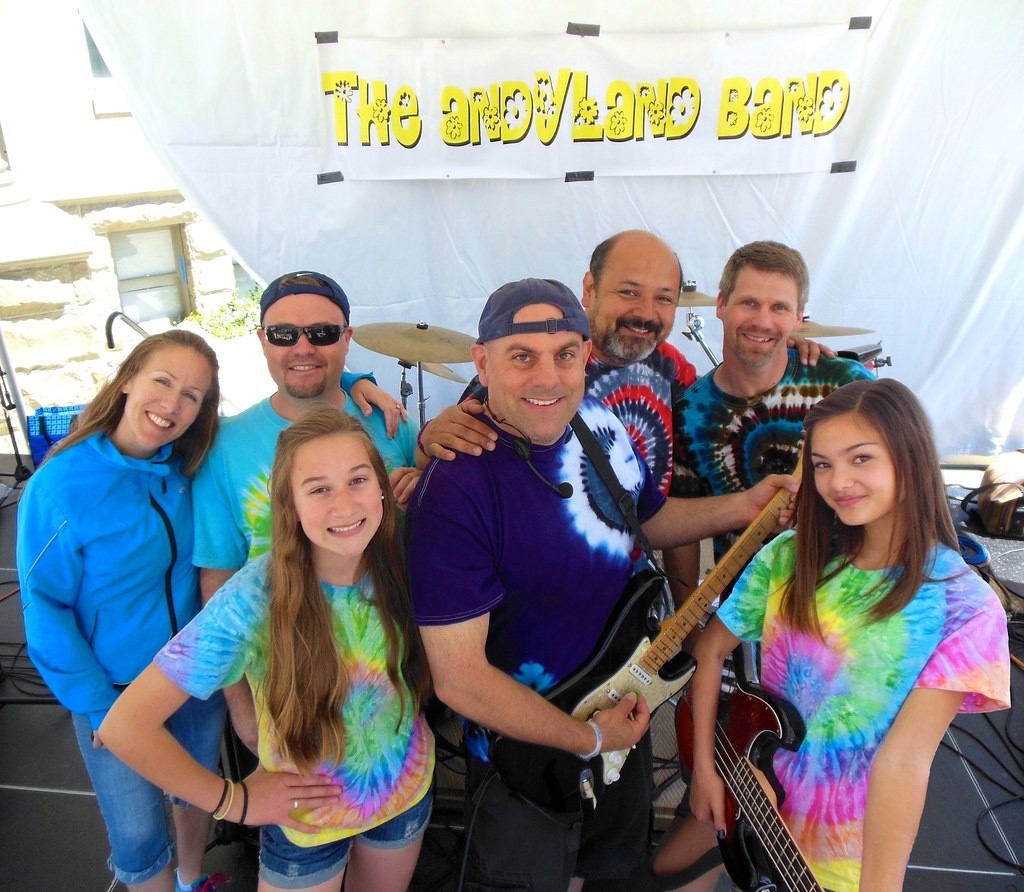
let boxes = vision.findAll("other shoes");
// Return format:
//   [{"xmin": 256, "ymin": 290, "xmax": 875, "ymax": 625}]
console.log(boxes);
[{"xmin": 174, "ymin": 869, "xmax": 242, "ymax": 892}]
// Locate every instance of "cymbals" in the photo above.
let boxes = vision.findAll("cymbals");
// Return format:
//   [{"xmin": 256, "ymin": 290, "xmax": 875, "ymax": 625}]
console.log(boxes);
[
  {"xmin": 675, "ymin": 291, "xmax": 719, "ymax": 309},
  {"xmin": 414, "ymin": 359, "xmax": 469, "ymax": 384},
  {"xmin": 800, "ymin": 319, "xmax": 874, "ymax": 338},
  {"xmin": 349, "ymin": 321, "xmax": 478, "ymax": 366}
]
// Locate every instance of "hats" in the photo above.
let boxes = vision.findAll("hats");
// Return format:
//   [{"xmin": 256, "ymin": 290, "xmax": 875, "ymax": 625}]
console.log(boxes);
[
  {"xmin": 475, "ymin": 278, "xmax": 590, "ymax": 343},
  {"xmin": 259, "ymin": 270, "xmax": 351, "ymax": 326}
]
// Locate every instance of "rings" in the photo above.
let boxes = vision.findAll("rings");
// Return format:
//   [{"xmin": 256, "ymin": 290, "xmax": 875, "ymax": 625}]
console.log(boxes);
[{"xmin": 394, "ymin": 404, "xmax": 400, "ymax": 409}]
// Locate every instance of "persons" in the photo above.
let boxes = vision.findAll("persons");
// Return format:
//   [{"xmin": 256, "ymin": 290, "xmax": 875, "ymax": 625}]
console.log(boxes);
[
  {"xmin": 98, "ymin": 407, "xmax": 436, "ymax": 892},
  {"xmin": 190, "ymin": 271, "xmax": 423, "ymax": 849},
  {"xmin": 402, "ymin": 280, "xmax": 803, "ymax": 892},
  {"xmin": 666, "ymin": 241, "xmax": 881, "ymax": 892},
  {"xmin": 414, "ymin": 231, "xmax": 839, "ymax": 475},
  {"xmin": 16, "ymin": 330, "xmax": 408, "ymax": 892},
  {"xmin": 650, "ymin": 378, "xmax": 1011, "ymax": 892}
]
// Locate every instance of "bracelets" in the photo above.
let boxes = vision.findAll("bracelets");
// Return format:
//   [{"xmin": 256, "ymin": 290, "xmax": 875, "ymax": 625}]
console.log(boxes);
[
  {"xmin": 417, "ymin": 419, "xmax": 435, "ymax": 459},
  {"xmin": 210, "ymin": 778, "xmax": 248, "ymax": 826},
  {"xmin": 573, "ymin": 721, "xmax": 603, "ymax": 763}
]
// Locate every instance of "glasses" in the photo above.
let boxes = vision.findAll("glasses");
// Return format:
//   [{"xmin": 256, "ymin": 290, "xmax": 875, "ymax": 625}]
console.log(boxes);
[{"xmin": 265, "ymin": 321, "xmax": 347, "ymax": 347}]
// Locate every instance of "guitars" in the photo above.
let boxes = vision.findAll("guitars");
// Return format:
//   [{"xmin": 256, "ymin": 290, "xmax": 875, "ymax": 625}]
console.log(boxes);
[
  {"xmin": 671, "ymin": 667, "xmax": 825, "ymax": 891},
  {"xmin": 487, "ymin": 445, "xmax": 816, "ymax": 824}
]
